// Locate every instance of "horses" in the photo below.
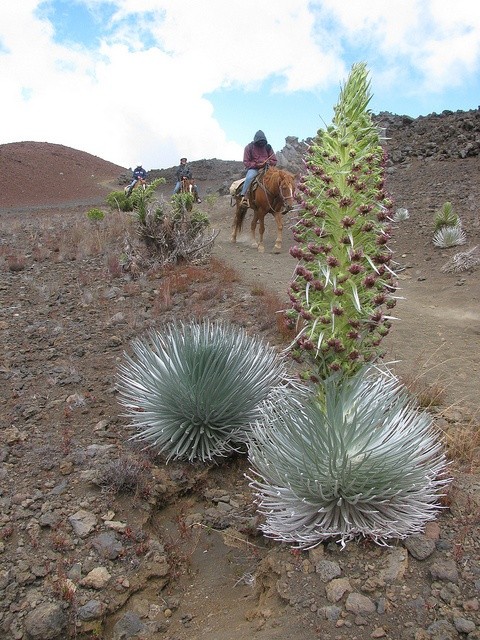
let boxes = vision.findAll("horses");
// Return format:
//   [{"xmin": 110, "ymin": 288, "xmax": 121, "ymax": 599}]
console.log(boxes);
[
  {"xmin": 124, "ymin": 178, "xmax": 149, "ymax": 198},
  {"xmin": 177, "ymin": 178, "xmax": 196, "ymax": 213},
  {"xmin": 230, "ymin": 166, "xmax": 301, "ymax": 254}
]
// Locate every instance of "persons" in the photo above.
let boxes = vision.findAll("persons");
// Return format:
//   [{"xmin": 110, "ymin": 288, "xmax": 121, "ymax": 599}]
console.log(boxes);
[
  {"xmin": 239, "ymin": 129, "xmax": 293, "ymax": 215},
  {"xmin": 170, "ymin": 157, "xmax": 201, "ymax": 204},
  {"xmin": 125, "ymin": 164, "xmax": 147, "ymax": 198}
]
